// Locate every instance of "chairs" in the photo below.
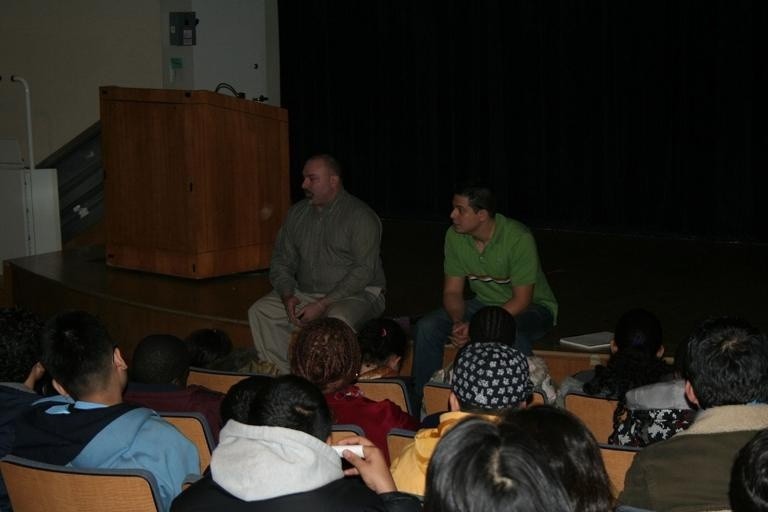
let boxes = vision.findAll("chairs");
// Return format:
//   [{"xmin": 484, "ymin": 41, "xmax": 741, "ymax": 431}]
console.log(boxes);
[
  {"xmin": 0, "ymin": 454, "xmax": 165, "ymax": 512},
  {"xmin": 387, "ymin": 428, "xmax": 416, "ymax": 465},
  {"xmin": 155, "ymin": 411, "xmax": 216, "ymax": 477},
  {"xmin": 564, "ymin": 392, "xmax": 620, "ymax": 443},
  {"xmin": 423, "ymin": 382, "xmax": 453, "ymax": 417},
  {"xmin": 186, "ymin": 366, "xmax": 272, "ymax": 396},
  {"xmin": 329, "ymin": 424, "xmax": 365, "ymax": 446},
  {"xmin": 598, "ymin": 444, "xmax": 643, "ymax": 501},
  {"xmin": 531, "ymin": 390, "xmax": 548, "ymax": 408},
  {"xmin": 353, "ymin": 379, "xmax": 413, "ymax": 417}
]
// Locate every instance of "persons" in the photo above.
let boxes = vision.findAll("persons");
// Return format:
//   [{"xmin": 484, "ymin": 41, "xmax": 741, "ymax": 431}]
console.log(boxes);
[
  {"xmin": 0, "ymin": 306, "xmax": 47, "ymax": 463},
  {"xmin": 615, "ymin": 319, "xmax": 768, "ymax": 512},
  {"xmin": 14, "ymin": 311, "xmax": 201, "ymax": 512},
  {"xmin": 423, "ymin": 405, "xmax": 622, "ymax": 512},
  {"xmin": 286, "ymin": 317, "xmax": 422, "ymax": 467},
  {"xmin": 246, "ymin": 153, "xmax": 387, "ymax": 375},
  {"xmin": 220, "ymin": 375, "xmax": 274, "ymax": 428},
  {"xmin": 170, "ymin": 373, "xmax": 423, "ymax": 512},
  {"xmin": 553, "ymin": 311, "xmax": 674, "ymax": 408},
  {"xmin": 390, "ymin": 341, "xmax": 533, "ymax": 497},
  {"xmin": 183, "ymin": 327, "xmax": 246, "ymax": 374},
  {"xmin": 407, "ymin": 175, "xmax": 566, "ymax": 393},
  {"xmin": 652, "ymin": 320, "xmax": 692, "ymax": 367},
  {"xmin": 126, "ymin": 333, "xmax": 228, "ymax": 445},
  {"xmin": 427, "ymin": 305, "xmax": 560, "ymax": 406},
  {"xmin": 606, "ymin": 355, "xmax": 699, "ymax": 449},
  {"xmin": 355, "ymin": 317, "xmax": 421, "ymax": 419}
]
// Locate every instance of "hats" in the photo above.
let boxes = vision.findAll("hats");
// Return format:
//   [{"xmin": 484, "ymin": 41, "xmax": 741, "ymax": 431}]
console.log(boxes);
[{"xmin": 453, "ymin": 345, "xmax": 535, "ymax": 407}]
